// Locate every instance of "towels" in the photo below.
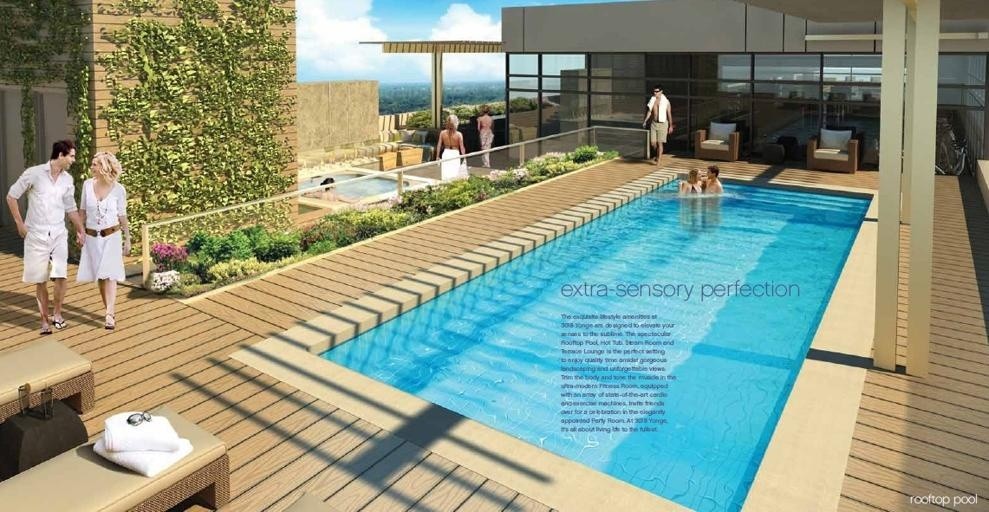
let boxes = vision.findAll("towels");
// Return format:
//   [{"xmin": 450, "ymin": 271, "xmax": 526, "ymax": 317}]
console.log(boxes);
[
  {"xmin": 647, "ymin": 93, "xmax": 670, "ymax": 123},
  {"xmin": 93, "ymin": 411, "xmax": 193, "ymax": 479}
]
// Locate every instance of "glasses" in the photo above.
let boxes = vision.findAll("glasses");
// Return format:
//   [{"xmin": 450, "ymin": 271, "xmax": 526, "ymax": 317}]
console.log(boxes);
[{"xmin": 127, "ymin": 411, "xmax": 152, "ymax": 426}]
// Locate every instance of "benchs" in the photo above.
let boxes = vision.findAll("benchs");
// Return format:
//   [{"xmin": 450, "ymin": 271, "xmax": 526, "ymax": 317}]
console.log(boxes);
[
  {"xmin": 0, "ymin": 405, "xmax": 230, "ymax": 512},
  {"xmin": 0, "ymin": 335, "xmax": 96, "ymax": 418}
]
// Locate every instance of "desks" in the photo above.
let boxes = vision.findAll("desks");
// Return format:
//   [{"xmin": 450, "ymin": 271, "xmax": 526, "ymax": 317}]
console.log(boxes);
[{"xmin": 0, "ymin": 398, "xmax": 88, "ymax": 470}]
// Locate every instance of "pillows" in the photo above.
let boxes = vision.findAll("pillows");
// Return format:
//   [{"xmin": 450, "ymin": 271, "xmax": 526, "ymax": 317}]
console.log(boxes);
[
  {"xmin": 707, "ymin": 121, "xmax": 737, "ymax": 140},
  {"xmin": 818, "ymin": 128, "xmax": 852, "ymax": 149}
]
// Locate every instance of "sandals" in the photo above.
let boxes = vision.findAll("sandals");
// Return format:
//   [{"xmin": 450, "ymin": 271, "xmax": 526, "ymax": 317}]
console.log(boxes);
[{"xmin": 105, "ymin": 313, "xmax": 116, "ymax": 329}]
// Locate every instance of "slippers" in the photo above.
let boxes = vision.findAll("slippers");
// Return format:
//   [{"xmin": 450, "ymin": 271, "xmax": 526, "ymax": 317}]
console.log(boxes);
[
  {"xmin": 53, "ymin": 320, "xmax": 67, "ymax": 329},
  {"xmin": 40, "ymin": 327, "xmax": 52, "ymax": 335}
]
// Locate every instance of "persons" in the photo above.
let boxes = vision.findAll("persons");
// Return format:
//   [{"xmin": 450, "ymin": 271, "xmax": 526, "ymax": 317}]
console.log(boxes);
[
  {"xmin": 476, "ymin": 104, "xmax": 495, "ymax": 168},
  {"xmin": 701, "ymin": 165, "xmax": 724, "ymax": 194},
  {"xmin": 703, "ymin": 197, "xmax": 721, "ymax": 231},
  {"xmin": 320, "ymin": 177, "xmax": 338, "ymax": 201},
  {"xmin": 436, "ymin": 114, "xmax": 468, "ymax": 181},
  {"xmin": 76, "ymin": 151, "xmax": 132, "ymax": 329},
  {"xmin": 642, "ymin": 83, "xmax": 673, "ymax": 168},
  {"xmin": 680, "ymin": 168, "xmax": 703, "ymax": 194},
  {"xmin": 681, "ymin": 198, "xmax": 703, "ymax": 235},
  {"xmin": 4, "ymin": 139, "xmax": 88, "ymax": 334}
]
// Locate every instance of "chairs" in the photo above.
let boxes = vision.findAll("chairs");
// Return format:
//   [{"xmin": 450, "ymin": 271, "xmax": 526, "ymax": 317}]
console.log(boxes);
[
  {"xmin": 694, "ymin": 121, "xmax": 741, "ymax": 162},
  {"xmin": 806, "ymin": 128, "xmax": 858, "ymax": 175}
]
